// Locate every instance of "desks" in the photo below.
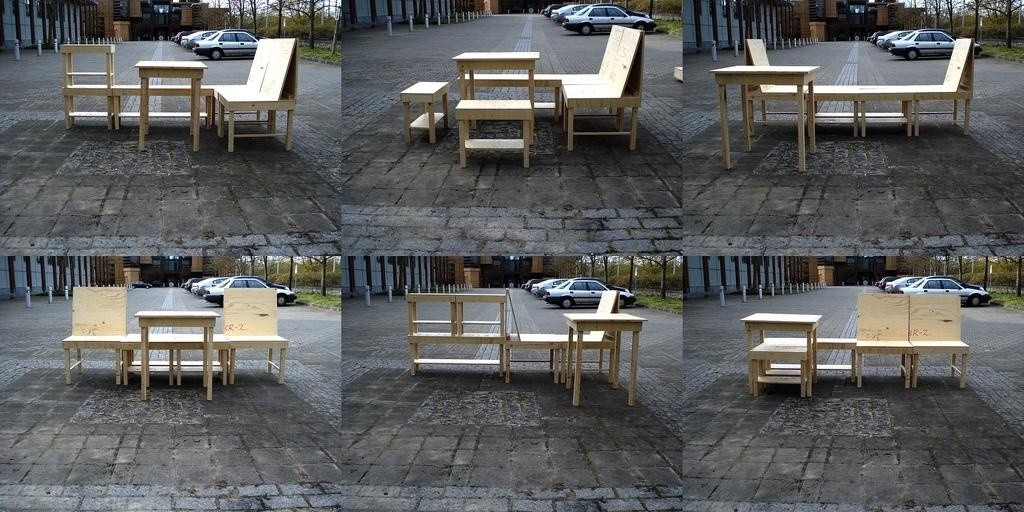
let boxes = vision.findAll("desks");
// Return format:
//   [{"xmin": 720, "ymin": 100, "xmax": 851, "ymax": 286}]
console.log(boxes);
[
  {"xmin": 132, "ymin": 59, "xmax": 209, "ymax": 147},
  {"xmin": 709, "ymin": 65, "xmax": 823, "ymax": 173},
  {"xmin": 563, "ymin": 311, "xmax": 647, "ymax": 406},
  {"xmin": 742, "ymin": 312, "xmax": 822, "ymax": 387},
  {"xmin": 135, "ymin": 308, "xmax": 217, "ymax": 398},
  {"xmin": 452, "ymin": 54, "xmax": 535, "ymax": 124}
]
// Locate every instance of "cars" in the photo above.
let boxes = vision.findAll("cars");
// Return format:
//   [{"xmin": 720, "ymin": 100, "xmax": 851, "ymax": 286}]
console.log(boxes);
[
  {"xmin": 202, "ymin": 278, "xmax": 296, "ymax": 307},
  {"xmin": 560, "ymin": 6, "xmax": 656, "ymax": 36},
  {"xmin": 127, "ymin": 280, "xmax": 152, "ymax": 289},
  {"xmin": 887, "ymin": 31, "xmax": 982, "ymax": 61},
  {"xmin": 898, "ymin": 278, "xmax": 992, "ymax": 307},
  {"xmin": 191, "ymin": 32, "xmax": 258, "ymax": 61},
  {"xmin": 180, "ymin": 276, "xmax": 289, "ymax": 297},
  {"xmin": 170, "ymin": 29, "xmax": 269, "ymax": 50},
  {"xmin": 875, "ymin": 276, "xmax": 984, "ymax": 294},
  {"xmin": 542, "ymin": 280, "xmax": 636, "ymax": 309},
  {"xmin": 540, "ymin": 3, "xmax": 649, "ymax": 26},
  {"xmin": 866, "ymin": 29, "xmax": 962, "ymax": 51},
  {"xmin": 520, "ymin": 277, "xmax": 630, "ymax": 298}
]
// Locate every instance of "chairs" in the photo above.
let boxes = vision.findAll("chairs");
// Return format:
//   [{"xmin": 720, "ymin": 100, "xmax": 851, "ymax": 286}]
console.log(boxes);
[
  {"xmin": 211, "ymin": 37, "xmax": 278, "ymax": 135},
  {"xmin": 222, "ymin": 287, "xmax": 288, "ymax": 383},
  {"xmin": 742, "ymin": 38, "xmax": 810, "ymax": 131},
  {"xmin": 218, "ymin": 37, "xmax": 300, "ymax": 152},
  {"xmin": 915, "ymin": 38, "xmax": 974, "ymax": 137},
  {"xmin": 558, "ymin": 29, "xmax": 642, "ymax": 154},
  {"xmin": 557, "ymin": 25, "xmax": 636, "ymax": 129},
  {"xmin": 908, "ymin": 296, "xmax": 970, "ymax": 390},
  {"xmin": 61, "ymin": 286, "xmax": 126, "ymax": 381},
  {"xmin": 505, "ymin": 287, "xmax": 559, "ymax": 384},
  {"xmin": 855, "ymin": 292, "xmax": 913, "ymax": 389},
  {"xmin": 557, "ymin": 290, "xmax": 616, "ymax": 383}
]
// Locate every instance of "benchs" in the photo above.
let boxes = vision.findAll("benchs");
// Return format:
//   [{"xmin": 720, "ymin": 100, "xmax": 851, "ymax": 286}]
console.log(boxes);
[
  {"xmin": 111, "ymin": 84, "xmax": 214, "ymax": 129},
  {"xmin": 408, "ymin": 332, "xmax": 504, "ymax": 379},
  {"xmin": 757, "ymin": 336, "xmax": 857, "ymax": 383},
  {"xmin": 455, "ymin": 75, "xmax": 559, "ymax": 114}
]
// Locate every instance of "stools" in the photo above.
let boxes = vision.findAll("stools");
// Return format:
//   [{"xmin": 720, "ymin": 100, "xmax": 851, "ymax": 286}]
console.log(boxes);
[
  {"xmin": 173, "ymin": 334, "xmax": 229, "ymax": 385},
  {"xmin": 456, "ymin": 294, "xmax": 505, "ymax": 336},
  {"xmin": 803, "ymin": 85, "xmax": 860, "ymax": 138},
  {"xmin": 755, "ymin": 339, "xmax": 808, "ymax": 393},
  {"xmin": 748, "ymin": 346, "xmax": 807, "ymax": 396},
  {"xmin": 62, "ymin": 84, "xmax": 116, "ymax": 129},
  {"xmin": 406, "ymin": 293, "xmax": 456, "ymax": 334},
  {"xmin": 122, "ymin": 333, "xmax": 174, "ymax": 387},
  {"xmin": 860, "ymin": 87, "xmax": 913, "ymax": 138},
  {"xmin": 398, "ymin": 81, "xmax": 448, "ymax": 143},
  {"xmin": 60, "ymin": 44, "xmax": 117, "ymax": 87},
  {"xmin": 457, "ymin": 97, "xmax": 533, "ymax": 164}
]
